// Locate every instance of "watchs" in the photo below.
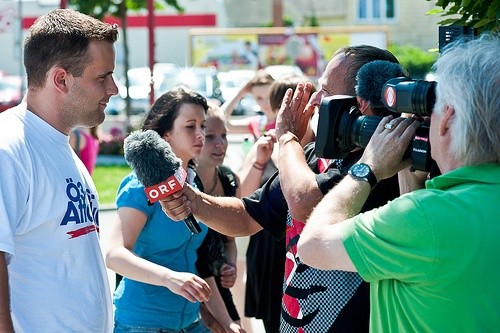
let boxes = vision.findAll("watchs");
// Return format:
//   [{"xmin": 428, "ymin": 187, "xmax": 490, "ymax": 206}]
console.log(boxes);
[{"xmin": 347, "ymin": 163, "xmax": 376, "ymax": 191}]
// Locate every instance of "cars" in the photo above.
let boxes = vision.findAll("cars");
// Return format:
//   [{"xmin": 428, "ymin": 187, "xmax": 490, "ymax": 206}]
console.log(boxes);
[{"xmin": 2, "ymin": 64, "xmax": 271, "ymax": 119}]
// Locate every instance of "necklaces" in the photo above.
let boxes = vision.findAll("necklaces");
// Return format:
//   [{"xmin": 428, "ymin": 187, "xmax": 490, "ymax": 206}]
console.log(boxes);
[{"xmin": 206, "ymin": 168, "xmax": 217, "ymax": 194}]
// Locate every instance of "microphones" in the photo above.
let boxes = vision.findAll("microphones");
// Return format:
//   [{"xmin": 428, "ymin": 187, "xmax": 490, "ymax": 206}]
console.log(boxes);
[
  {"xmin": 124, "ymin": 129, "xmax": 202, "ymax": 234},
  {"xmin": 357, "ymin": 60, "xmax": 413, "ymax": 111}
]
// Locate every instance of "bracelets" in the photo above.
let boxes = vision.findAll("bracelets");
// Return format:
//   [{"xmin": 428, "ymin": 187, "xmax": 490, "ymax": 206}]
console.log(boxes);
[
  {"xmin": 252, "ymin": 161, "xmax": 265, "ymax": 171},
  {"xmin": 227, "ymin": 262, "xmax": 236, "ymax": 269}
]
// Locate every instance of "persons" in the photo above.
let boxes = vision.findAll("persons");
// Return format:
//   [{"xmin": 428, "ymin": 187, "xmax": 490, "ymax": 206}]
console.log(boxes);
[
  {"xmin": 69, "ymin": 34, "xmax": 500, "ymax": 333},
  {"xmin": 0, "ymin": 10, "xmax": 118, "ymax": 333}
]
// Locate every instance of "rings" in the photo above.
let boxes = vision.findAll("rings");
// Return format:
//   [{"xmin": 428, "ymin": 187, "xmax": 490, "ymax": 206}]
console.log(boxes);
[
  {"xmin": 384, "ymin": 124, "xmax": 394, "ymax": 130},
  {"xmin": 263, "ymin": 133, "xmax": 268, "ymax": 138}
]
